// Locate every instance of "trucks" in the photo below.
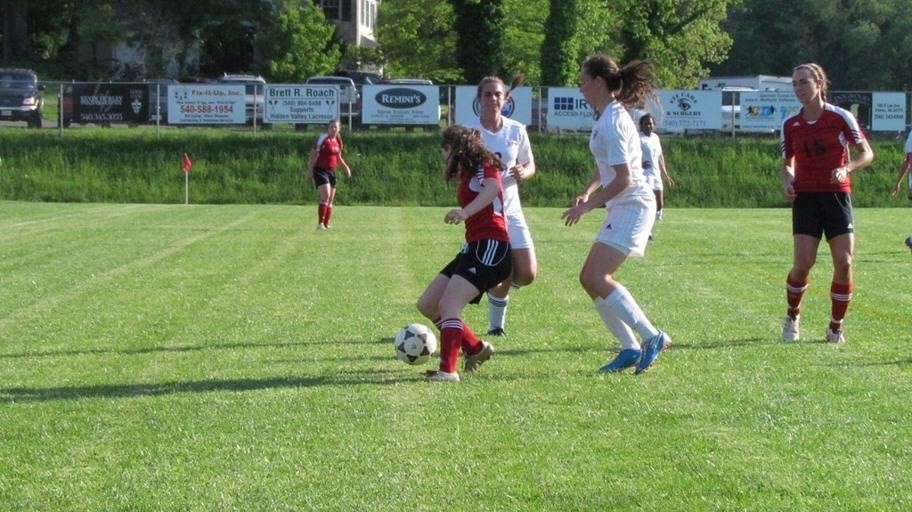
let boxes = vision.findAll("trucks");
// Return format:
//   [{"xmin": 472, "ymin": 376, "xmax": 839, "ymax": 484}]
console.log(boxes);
[{"xmin": 698, "ymin": 74, "xmax": 794, "ymax": 92}]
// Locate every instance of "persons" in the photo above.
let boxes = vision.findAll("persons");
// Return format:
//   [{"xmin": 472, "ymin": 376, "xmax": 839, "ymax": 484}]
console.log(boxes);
[
  {"xmin": 639, "ymin": 113, "xmax": 674, "ymax": 241},
  {"xmin": 417, "ymin": 125, "xmax": 513, "ymax": 382},
  {"xmin": 466, "ymin": 71, "xmax": 538, "ymax": 337},
  {"xmin": 778, "ymin": 61, "xmax": 873, "ymax": 345},
  {"xmin": 560, "ymin": 55, "xmax": 663, "ymax": 373},
  {"xmin": 889, "ymin": 127, "xmax": 912, "ymax": 253},
  {"xmin": 305, "ymin": 120, "xmax": 352, "ymax": 230}
]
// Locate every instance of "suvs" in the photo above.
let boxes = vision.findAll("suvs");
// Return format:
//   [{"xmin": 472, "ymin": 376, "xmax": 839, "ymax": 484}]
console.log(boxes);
[
  {"xmin": 333, "ymin": 71, "xmax": 382, "ymax": 94},
  {"xmin": 57, "ymin": 79, "xmax": 111, "ymax": 128},
  {"xmin": 379, "ymin": 79, "xmax": 441, "ymax": 133},
  {"xmin": 219, "ymin": 71, "xmax": 272, "ymax": 130},
  {"xmin": 129, "ymin": 74, "xmax": 181, "ymax": 122},
  {"xmin": 0, "ymin": 68, "xmax": 47, "ymax": 129},
  {"xmin": 717, "ymin": 86, "xmax": 759, "ymax": 130}
]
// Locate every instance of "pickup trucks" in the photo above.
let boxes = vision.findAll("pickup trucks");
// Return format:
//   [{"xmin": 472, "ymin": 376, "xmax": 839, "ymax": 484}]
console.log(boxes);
[{"xmin": 294, "ymin": 76, "xmax": 360, "ymax": 130}]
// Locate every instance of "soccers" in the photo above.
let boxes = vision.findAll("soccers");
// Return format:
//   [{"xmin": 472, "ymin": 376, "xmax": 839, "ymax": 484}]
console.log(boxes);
[{"xmin": 395, "ymin": 323, "xmax": 436, "ymax": 366}]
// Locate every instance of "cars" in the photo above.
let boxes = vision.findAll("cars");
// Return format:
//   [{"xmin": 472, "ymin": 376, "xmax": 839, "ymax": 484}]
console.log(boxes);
[
  {"xmin": 446, "ymin": 97, "xmax": 547, "ymax": 131},
  {"xmin": 178, "ymin": 77, "xmax": 218, "ymax": 84}
]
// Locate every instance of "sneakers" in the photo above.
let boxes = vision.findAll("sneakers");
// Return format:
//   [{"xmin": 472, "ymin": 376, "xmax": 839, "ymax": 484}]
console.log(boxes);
[
  {"xmin": 782, "ymin": 314, "xmax": 800, "ymax": 342},
  {"xmin": 826, "ymin": 328, "xmax": 845, "ymax": 342},
  {"xmin": 461, "ymin": 339, "xmax": 494, "ymax": 372},
  {"xmin": 598, "ymin": 349, "xmax": 642, "ymax": 371},
  {"xmin": 424, "ymin": 370, "xmax": 460, "ymax": 382},
  {"xmin": 317, "ymin": 223, "xmax": 326, "ymax": 229},
  {"xmin": 488, "ymin": 328, "xmax": 505, "ymax": 337},
  {"xmin": 635, "ymin": 329, "xmax": 672, "ymax": 374}
]
